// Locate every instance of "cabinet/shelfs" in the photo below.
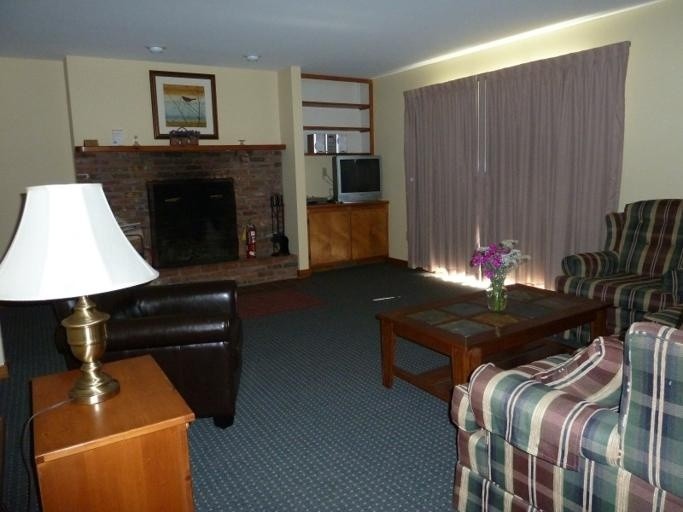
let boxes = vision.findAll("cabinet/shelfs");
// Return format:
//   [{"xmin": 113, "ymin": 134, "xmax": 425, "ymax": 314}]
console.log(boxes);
[
  {"xmin": 302, "ymin": 72, "xmax": 375, "ymax": 156},
  {"xmin": 306, "ymin": 200, "xmax": 390, "ymax": 273},
  {"xmin": 29, "ymin": 353, "xmax": 198, "ymax": 512}
]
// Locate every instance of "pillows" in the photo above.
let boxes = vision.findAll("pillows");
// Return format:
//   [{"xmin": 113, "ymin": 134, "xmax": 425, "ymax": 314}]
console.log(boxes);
[{"xmin": 530, "ymin": 335, "xmax": 624, "ymax": 413}]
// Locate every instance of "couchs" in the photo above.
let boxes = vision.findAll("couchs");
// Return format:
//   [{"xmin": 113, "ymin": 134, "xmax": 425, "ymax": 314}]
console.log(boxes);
[
  {"xmin": 554, "ymin": 198, "xmax": 682, "ymax": 342},
  {"xmin": 50, "ymin": 278, "xmax": 245, "ymax": 429},
  {"xmin": 449, "ymin": 320, "xmax": 683, "ymax": 512}
]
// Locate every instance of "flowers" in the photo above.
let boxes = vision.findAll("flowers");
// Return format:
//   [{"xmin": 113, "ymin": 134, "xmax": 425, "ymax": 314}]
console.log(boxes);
[{"xmin": 468, "ymin": 239, "xmax": 532, "ymax": 280}]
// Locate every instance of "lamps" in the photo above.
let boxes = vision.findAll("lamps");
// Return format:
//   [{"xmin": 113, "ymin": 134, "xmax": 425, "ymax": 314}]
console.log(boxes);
[{"xmin": 1, "ymin": 182, "xmax": 161, "ymax": 408}]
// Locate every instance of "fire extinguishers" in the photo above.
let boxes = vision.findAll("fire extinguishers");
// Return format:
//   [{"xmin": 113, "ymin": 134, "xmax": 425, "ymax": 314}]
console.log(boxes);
[{"xmin": 247, "ymin": 216, "xmax": 256, "ymax": 258}]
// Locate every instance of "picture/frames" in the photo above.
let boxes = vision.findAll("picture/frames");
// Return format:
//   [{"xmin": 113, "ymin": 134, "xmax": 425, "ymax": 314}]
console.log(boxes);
[{"xmin": 146, "ymin": 68, "xmax": 220, "ymax": 141}]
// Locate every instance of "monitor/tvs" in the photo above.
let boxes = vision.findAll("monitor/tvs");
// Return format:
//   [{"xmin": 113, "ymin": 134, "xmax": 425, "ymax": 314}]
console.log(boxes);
[{"xmin": 335, "ymin": 154, "xmax": 382, "ymax": 202}]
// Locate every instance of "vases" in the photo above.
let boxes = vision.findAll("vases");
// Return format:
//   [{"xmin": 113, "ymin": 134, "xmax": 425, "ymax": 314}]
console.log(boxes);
[{"xmin": 484, "ymin": 277, "xmax": 510, "ymax": 313}]
